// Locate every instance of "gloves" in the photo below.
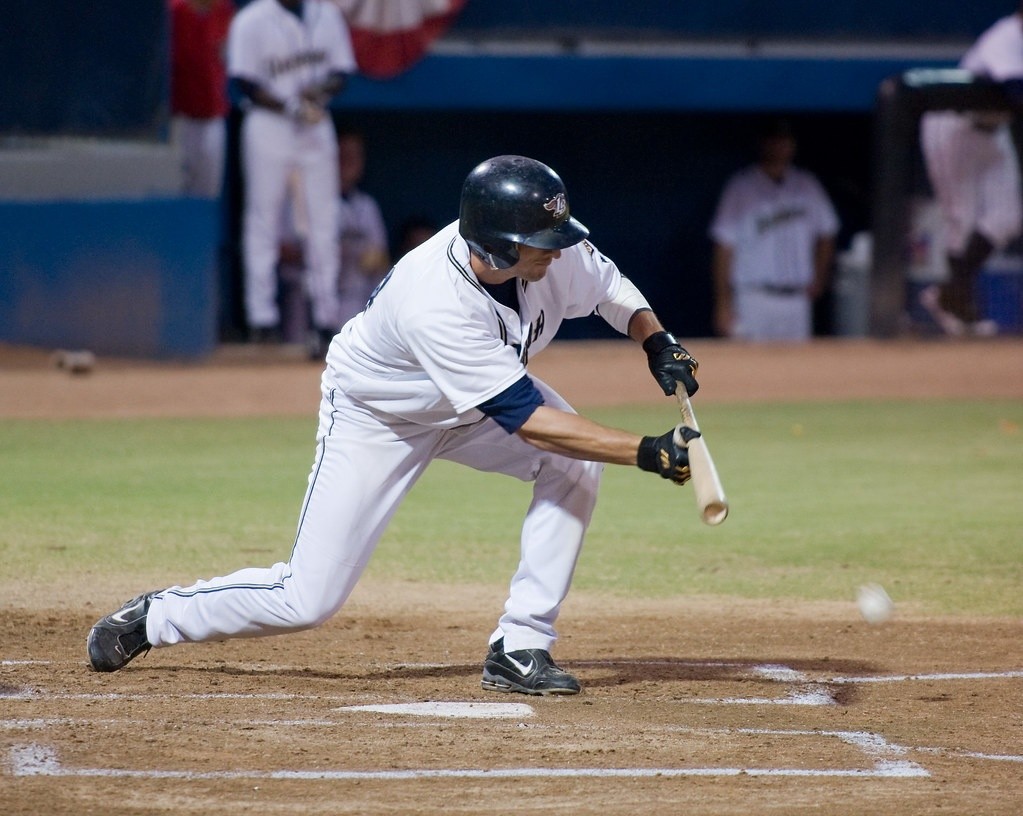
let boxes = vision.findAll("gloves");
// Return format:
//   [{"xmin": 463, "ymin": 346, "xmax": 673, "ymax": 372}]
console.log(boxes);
[
  {"xmin": 642, "ymin": 331, "xmax": 700, "ymax": 398},
  {"xmin": 637, "ymin": 425, "xmax": 701, "ymax": 485}
]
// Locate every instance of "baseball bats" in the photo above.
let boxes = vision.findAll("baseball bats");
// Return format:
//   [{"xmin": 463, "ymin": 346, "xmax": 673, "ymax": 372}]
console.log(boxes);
[{"xmin": 674, "ymin": 379, "xmax": 729, "ymax": 527}]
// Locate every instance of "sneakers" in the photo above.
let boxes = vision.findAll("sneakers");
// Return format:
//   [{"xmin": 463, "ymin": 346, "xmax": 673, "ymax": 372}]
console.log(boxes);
[
  {"xmin": 87, "ymin": 589, "xmax": 152, "ymax": 672},
  {"xmin": 481, "ymin": 638, "xmax": 581, "ymax": 695}
]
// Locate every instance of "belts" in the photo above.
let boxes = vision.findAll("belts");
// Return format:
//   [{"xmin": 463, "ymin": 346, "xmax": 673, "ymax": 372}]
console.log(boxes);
[{"xmin": 764, "ymin": 285, "xmax": 800, "ymax": 297}]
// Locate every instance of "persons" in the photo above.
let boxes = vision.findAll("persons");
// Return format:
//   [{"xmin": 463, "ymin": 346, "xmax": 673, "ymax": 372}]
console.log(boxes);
[
  {"xmin": 225, "ymin": 0, "xmax": 357, "ymax": 361},
  {"xmin": 302, "ymin": 134, "xmax": 390, "ymax": 346},
  {"xmin": 87, "ymin": 156, "xmax": 700, "ymax": 692},
  {"xmin": 919, "ymin": 14, "xmax": 1023, "ymax": 336},
  {"xmin": 168, "ymin": 0, "xmax": 232, "ymax": 198},
  {"xmin": 710, "ymin": 122, "xmax": 838, "ymax": 340}
]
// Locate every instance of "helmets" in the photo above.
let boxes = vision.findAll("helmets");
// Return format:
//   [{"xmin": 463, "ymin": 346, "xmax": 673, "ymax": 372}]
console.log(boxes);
[{"xmin": 459, "ymin": 154, "xmax": 590, "ymax": 268}]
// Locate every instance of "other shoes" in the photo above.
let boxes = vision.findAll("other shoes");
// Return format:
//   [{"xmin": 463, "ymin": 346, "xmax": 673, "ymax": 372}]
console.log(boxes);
[
  {"xmin": 307, "ymin": 331, "xmax": 328, "ymax": 360},
  {"xmin": 249, "ymin": 327, "xmax": 282, "ymax": 344}
]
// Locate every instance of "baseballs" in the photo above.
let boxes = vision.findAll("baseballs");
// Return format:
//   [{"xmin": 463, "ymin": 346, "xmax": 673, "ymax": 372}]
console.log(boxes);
[{"xmin": 854, "ymin": 582, "xmax": 898, "ymax": 627}]
